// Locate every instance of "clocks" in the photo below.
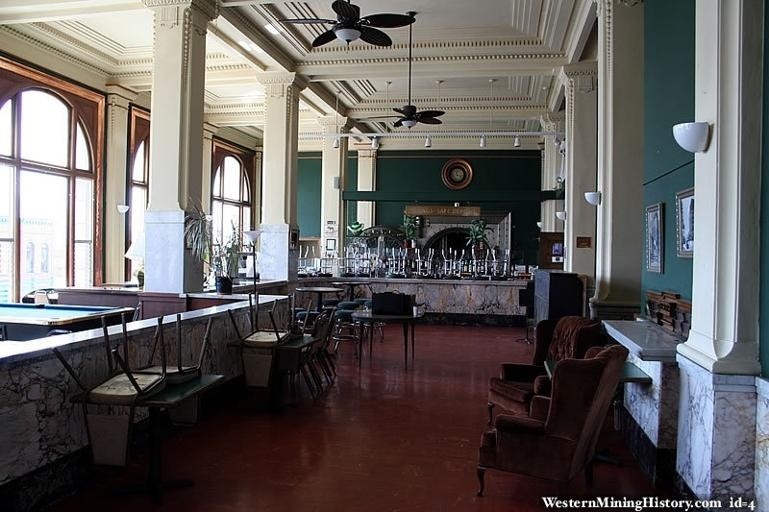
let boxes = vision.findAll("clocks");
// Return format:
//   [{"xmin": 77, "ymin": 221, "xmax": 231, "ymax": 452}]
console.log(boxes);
[{"xmin": 441, "ymin": 159, "xmax": 474, "ymax": 191}]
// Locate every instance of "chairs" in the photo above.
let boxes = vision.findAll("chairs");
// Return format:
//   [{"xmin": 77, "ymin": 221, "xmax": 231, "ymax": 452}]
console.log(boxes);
[
  {"xmin": 524, "ymin": 280, "xmax": 536, "ymax": 343},
  {"xmin": 52, "ymin": 292, "xmax": 337, "ymax": 470},
  {"xmin": 476, "ymin": 345, "xmax": 633, "ymax": 496},
  {"xmin": 488, "ymin": 316, "xmax": 603, "ymax": 422}
]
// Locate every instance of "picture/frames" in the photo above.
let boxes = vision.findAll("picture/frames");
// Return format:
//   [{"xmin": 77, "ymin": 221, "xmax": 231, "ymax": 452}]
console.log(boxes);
[
  {"xmin": 645, "ymin": 202, "xmax": 666, "ymax": 274},
  {"xmin": 674, "ymin": 188, "xmax": 694, "ymax": 258}
]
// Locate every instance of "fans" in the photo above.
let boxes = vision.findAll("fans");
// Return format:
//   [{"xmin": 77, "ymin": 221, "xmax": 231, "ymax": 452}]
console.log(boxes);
[
  {"xmin": 278, "ymin": 0, "xmax": 416, "ymax": 47},
  {"xmin": 395, "ymin": 11, "xmax": 444, "ymax": 128}
]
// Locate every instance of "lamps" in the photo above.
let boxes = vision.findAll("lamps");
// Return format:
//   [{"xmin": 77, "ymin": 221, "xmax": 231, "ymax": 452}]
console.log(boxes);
[
  {"xmin": 332, "ymin": 78, "xmax": 552, "ymax": 150},
  {"xmin": 536, "ymin": 192, "xmax": 600, "ymax": 228},
  {"xmin": 672, "ymin": 122, "xmax": 710, "ymax": 153},
  {"xmin": 117, "ymin": 205, "xmax": 129, "ymax": 214}
]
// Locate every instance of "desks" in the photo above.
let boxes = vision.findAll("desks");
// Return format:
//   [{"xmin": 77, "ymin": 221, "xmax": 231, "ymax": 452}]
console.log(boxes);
[{"xmin": 542, "ymin": 359, "xmax": 651, "ymax": 468}]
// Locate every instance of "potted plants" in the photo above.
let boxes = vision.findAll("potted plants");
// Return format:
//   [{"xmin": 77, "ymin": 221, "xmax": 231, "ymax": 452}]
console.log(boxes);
[
  {"xmin": 184, "ymin": 195, "xmax": 243, "ymax": 295},
  {"xmin": 402, "ymin": 211, "xmax": 418, "ymax": 258},
  {"xmin": 466, "ymin": 219, "xmax": 494, "ymax": 259}
]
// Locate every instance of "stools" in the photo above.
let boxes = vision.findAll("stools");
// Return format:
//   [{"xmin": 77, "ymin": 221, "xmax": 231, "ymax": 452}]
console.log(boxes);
[{"xmin": 294, "ymin": 246, "xmax": 508, "ymax": 281}]
